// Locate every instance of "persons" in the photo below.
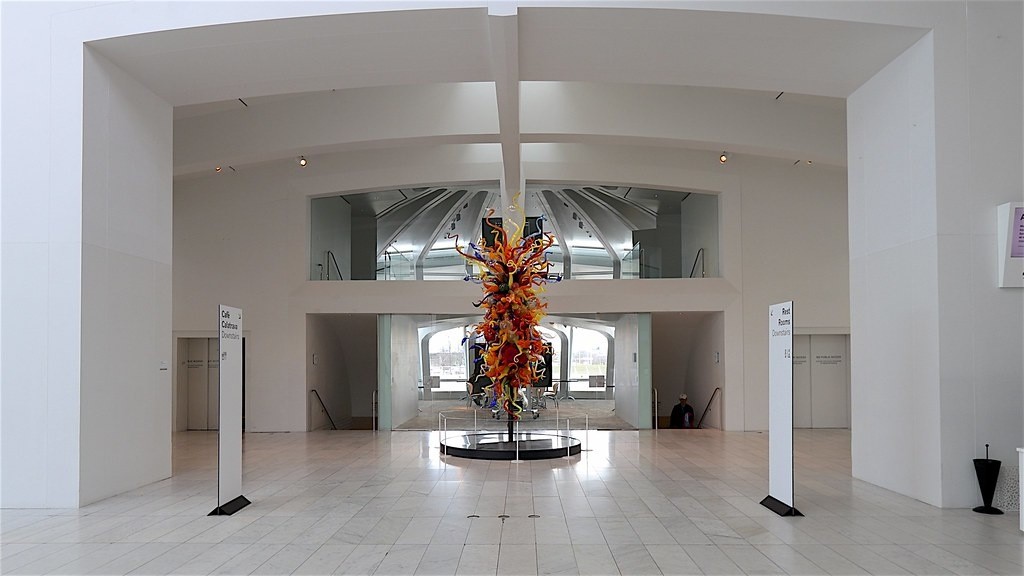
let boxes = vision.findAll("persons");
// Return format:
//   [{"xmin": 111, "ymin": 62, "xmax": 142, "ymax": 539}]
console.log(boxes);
[{"xmin": 669, "ymin": 393, "xmax": 695, "ymax": 429}]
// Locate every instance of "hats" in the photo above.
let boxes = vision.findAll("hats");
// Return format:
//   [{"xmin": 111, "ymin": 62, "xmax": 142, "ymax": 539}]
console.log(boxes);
[{"xmin": 679, "ymin": 393, "xmax": 688, "ymax": 400}]
